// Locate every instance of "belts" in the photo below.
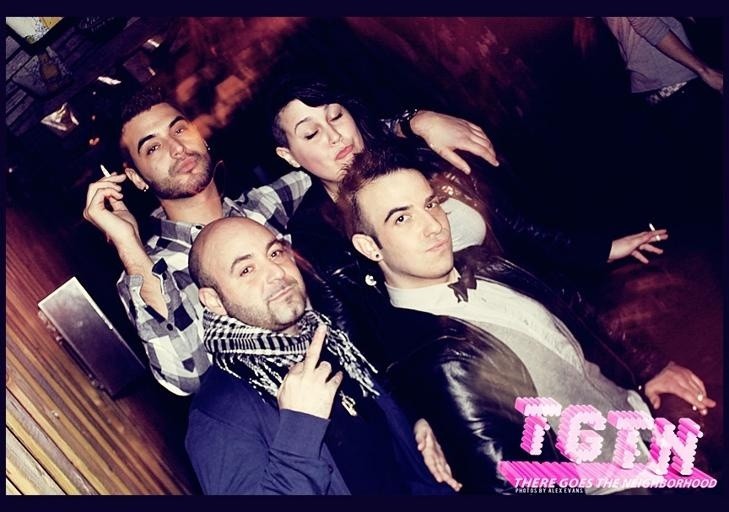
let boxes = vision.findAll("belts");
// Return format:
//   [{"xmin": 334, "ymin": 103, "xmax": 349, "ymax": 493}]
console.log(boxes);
[{"xmin": 646, "ymin": 82, "xmax": 687, "ymax": 104}]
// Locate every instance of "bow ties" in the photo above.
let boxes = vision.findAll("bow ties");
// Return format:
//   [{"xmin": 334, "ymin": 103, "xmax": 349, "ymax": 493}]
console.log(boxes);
[{"xmin": 448, "ymin": 265, "xmax": 477, "ymax": 303}]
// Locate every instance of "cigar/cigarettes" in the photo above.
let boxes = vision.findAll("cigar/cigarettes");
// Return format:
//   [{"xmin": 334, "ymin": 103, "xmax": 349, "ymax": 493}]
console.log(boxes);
[
  {"xmin": 692, "ymin": 392, "xmax": 704, "ymax": 411},
  {"xmin": 647, "ymin": 222, "xmax": 662, "ymax": 242}
]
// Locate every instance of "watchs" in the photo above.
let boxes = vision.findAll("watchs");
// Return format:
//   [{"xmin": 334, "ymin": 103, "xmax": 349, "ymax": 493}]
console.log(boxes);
[{"xmin": 396, "ymin": 107, "xmax": 420, "ymax": 137}]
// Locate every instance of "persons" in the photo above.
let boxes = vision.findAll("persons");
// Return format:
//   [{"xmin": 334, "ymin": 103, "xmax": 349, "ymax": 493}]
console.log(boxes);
[
  {"xmin": 182, "ymin": 217, "xmax": 476, "ymax": 497},
  {"xmin": 604, "ymin": 17, "xmax": 724, "ymax": 176},
  {"xmin": 265, "ymin": 68, "xmax": 670, "ymax": 373},
  {"xmin": 82, "ymin": 86, "xmax": 502, "ymax": 398},
  {"xmin": 332, "ymin": 146, "xmax": 718, "ymax": 494},
  {"xmin": 100, "ymin": 164, "xmax": 110, "ymax": 178}
]
